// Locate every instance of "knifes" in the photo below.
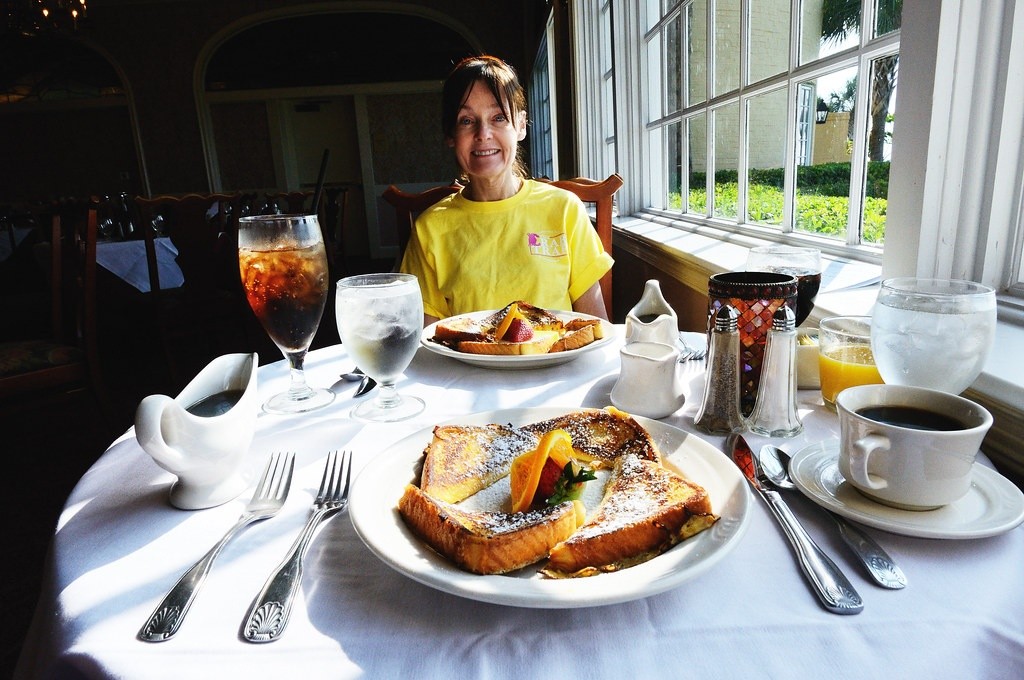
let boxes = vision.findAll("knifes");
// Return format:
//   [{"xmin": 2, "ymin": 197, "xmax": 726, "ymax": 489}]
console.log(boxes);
[
  {"xmin": 352, "ymin": 376, "xmax": 377, "ymax": 399},
  {"xmin": 724, "ymin": 432, "xmax": 866, "ymax": 616}
]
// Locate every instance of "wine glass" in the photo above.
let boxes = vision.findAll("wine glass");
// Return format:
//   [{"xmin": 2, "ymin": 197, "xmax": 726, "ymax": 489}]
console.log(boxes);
[
  {"xmin": 237, "ymin": 213, "xmax": 336, "ymax": 415},
  {"xmin": 336, "ymin": 272, "xmax": 426, "ymax": 423}
]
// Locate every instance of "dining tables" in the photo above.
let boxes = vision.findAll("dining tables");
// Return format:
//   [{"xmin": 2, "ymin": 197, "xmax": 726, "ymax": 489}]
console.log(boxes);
[{"xmin": 5, "ymin": 330, "xmax": 979, "ymax": 680}]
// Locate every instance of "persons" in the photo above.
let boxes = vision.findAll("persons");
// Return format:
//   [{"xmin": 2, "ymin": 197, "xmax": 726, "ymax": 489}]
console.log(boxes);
[{"xmin": 399, "ymin": 47, "xmax": 615, "ymax": 322}]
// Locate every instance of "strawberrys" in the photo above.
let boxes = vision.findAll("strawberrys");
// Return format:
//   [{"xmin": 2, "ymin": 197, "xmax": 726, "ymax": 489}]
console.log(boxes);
[
  {"xmin": 540, "ymin": 457, "xmax": 598, "ymax": 506},
  {"xmin": 503, "ymin": 318, "xmax": 534, "ymax": 343}
]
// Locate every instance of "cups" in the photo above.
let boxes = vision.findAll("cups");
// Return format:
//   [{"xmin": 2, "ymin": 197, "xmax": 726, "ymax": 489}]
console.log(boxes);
[
  {"xmin": 819, "ymin": 316, "xmax": 886, "ymax": 413},
  {"xmin": 610, "ymin": 341, "xmax": 685, "ymax": 420},
  {"xmin": 745, "ymin": 246, "xmax": 822, "ymax": 329},
  {"xmin": 151, "ymin": 204, "xmax": 170, "ymax": 237},
  {"xmin": 707, "ymin": 271, "xmax": 799, "ymax": 417},
  {"xmin": 870, "ymin": 277, "xmax": 999, "ymax": 397},
  {"xmin": 797, "ymin": 328, "xmax": 820, "ymax": 390},
  {"xmin": 835, "ymin": 383, "xmax": 994, "ymax": 512}
]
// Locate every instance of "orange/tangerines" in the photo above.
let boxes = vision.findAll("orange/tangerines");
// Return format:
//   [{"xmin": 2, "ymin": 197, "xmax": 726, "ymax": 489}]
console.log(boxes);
[
  {"xmin": 510, "ymin": 429, "xmax": 577, "ymax": 515},
  {"xmin": 493, "ymin": 303, "xmax": 530, "ymax": 339}
]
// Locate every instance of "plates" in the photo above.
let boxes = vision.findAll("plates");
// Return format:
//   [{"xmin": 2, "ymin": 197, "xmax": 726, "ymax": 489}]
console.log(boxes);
[
  {"xmin": 788, "ymin": 440, "xmax": 1024, "ymax": 541},
  {"xmin": 420, "ymin": 309, "xmax": 619, "ymax": 371},
  {"xmin": 346, "ymin": 405, "xmax": 753, "ymax": 610}
]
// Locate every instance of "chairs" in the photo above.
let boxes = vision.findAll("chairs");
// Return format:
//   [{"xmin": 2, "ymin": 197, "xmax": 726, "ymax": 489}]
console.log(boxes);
[
  {"xmin": 0, "ymin": 189, "xmax": 354, "ymax": 451},
  {"xmin": 377, "ymin": 171, "xmax": 623, "ymax": 323}
]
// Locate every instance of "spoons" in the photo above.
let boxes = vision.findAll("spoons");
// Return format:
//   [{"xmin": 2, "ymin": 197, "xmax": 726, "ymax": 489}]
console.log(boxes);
[
  {"xmin": 339, "ymin": 366, "xmax": 366, "ymax": 381},
  {"xmin": 759, "ymin": 443, "xmax": 908, "ymax": 590}
]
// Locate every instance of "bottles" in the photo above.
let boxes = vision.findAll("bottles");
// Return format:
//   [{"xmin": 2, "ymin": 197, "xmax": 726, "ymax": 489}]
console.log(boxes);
[
  {"xmin": 693, "ymin": 304, "xmax": 750, "ymax": 437},
  {"xmin": 98, "ymin": 191, "xmax": 137, "ymax": 242},
  {"xmin": 744, "ymin": 305, "xmax": 803, "ymax": 438}
]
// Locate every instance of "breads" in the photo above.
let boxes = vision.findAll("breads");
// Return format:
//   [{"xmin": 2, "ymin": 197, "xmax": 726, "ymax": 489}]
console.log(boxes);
[
  {"xmin": 400, "ymin": 406, "xmax": 713, "ymax": 578},
  {"xmin": 431, "ymin": 301, "xmax": 603, "ymax": 357}
]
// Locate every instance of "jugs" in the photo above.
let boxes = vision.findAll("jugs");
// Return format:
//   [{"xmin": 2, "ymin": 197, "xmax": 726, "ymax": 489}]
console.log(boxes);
[
  {"xmin": 625, "ymin": 279, "xmax": 680, "ymax": 349},
  {"xmin": 133, "ymin": 351, "xmax": 259, "ymax": 510}
]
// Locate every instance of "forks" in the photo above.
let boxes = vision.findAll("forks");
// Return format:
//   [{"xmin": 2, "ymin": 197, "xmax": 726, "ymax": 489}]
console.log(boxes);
[
  {"xmin": 679, "ymin": 329, "xmax": 707, "ymax": 363},
  {"xmin": 244, "ymin": 449, "xmax": 354, "ymax": 643},
  {"xmin": 138, "ymin": 450, "xmax": 297, "ymax": 643}
]
